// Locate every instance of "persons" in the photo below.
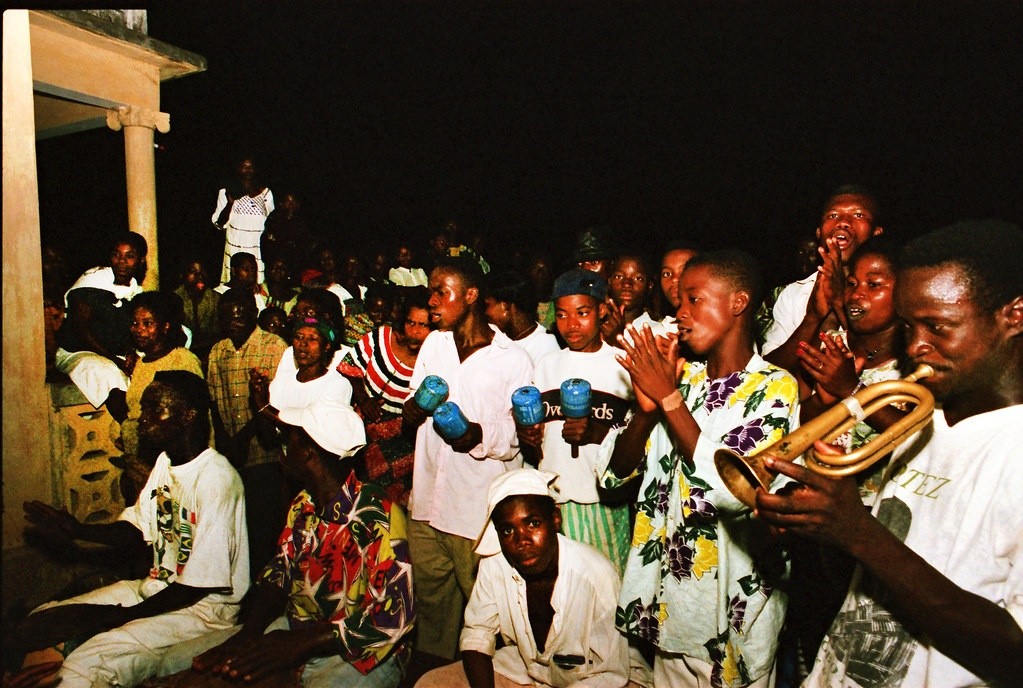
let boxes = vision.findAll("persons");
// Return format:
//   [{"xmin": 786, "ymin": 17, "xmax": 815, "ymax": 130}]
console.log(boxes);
[
  {"xmin": 210, "ymin": 159, "xmax": 277, "ymax": 290},
  {"xmin": 0, "ymin": 186, "xmax": 1023, "ymax": 688}
]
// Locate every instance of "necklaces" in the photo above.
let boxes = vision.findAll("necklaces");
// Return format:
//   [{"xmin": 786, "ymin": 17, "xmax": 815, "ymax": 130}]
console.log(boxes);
[{"xmin": 864, "ymin": 342, "xmax": 891, "ymax": 362}]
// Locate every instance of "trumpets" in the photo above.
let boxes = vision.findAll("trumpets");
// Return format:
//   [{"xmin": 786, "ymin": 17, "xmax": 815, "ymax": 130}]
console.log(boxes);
[{"xmin": 712, "ymin": 361, "xmax": 940, "ymax": 514}]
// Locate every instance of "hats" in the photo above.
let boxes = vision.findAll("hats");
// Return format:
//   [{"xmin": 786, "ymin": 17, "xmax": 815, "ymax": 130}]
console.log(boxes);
[
  {"xmin": 469, "ymin": 467, "xmax": 560, "ymax": 556},
  {"xmin": 569, "ymin": 225, "xmax": 613, "ymax": 264},
  {"xmin": 550, "ymin": 268, "xmax": 607, "ymax": 304},
  {"xmin": 280, "ymin": 400, "xmax": 367, "ymax": 458}
]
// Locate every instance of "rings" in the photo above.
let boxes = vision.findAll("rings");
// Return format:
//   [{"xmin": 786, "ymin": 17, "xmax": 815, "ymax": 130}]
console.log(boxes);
[{"xmin": 817, "ymin": 363, "xmax": 823, "ymax": 371}]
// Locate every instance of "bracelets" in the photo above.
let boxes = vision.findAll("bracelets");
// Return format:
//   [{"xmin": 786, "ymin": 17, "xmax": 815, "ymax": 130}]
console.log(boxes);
[
  {"xmin": 654, "ymin": 389, "xmax": 683, "ymax": 412},
  {"xmin": 258, "ymin": 404, "xmax": 269, "ymax": 413}
]
